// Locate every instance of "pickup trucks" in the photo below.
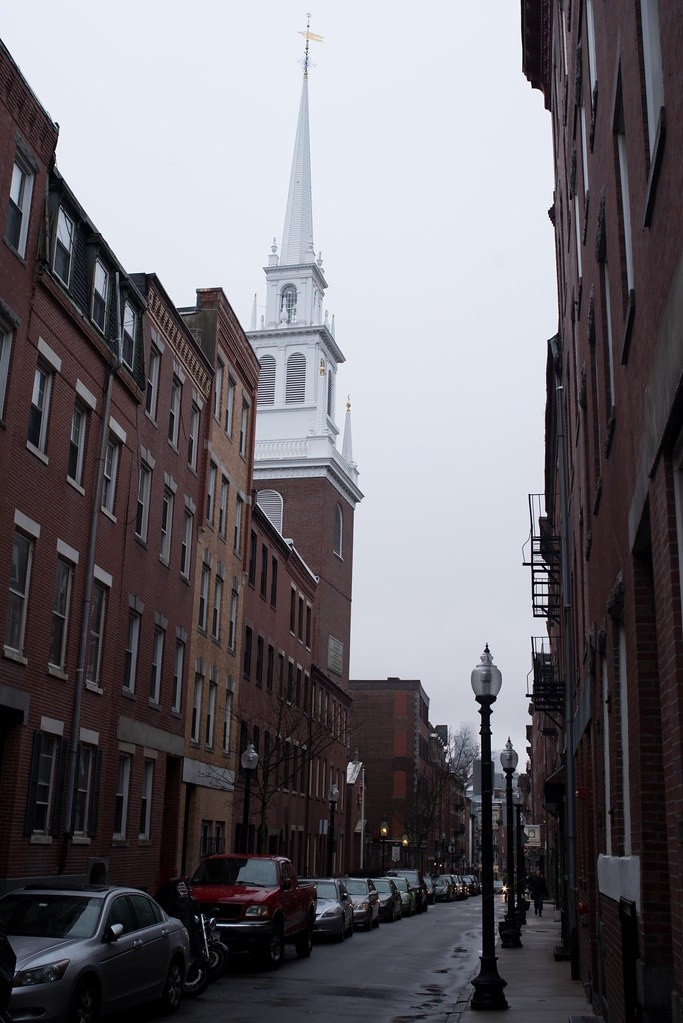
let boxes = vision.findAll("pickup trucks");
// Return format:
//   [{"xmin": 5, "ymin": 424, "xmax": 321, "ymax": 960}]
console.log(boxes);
[{"xmin": 180, "ymin": 854, "xmax": 318, "ymax": 969}]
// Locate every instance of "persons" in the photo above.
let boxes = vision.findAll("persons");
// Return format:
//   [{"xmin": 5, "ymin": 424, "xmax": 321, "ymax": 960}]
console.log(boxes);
[
  {"xmin": 503, "ymin": 875, "xmax": 509, "ymax": 902},
  {"xmin": 0, "ymin": 923, "xmax": 19, "ymax": 1023},
  {"xmin": 528, "ymin": 870, "xmax": 548, "ymax": 917}
]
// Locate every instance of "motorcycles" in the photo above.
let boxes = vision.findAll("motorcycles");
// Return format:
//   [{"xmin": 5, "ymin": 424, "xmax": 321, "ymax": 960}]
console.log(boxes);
[
  {"xmin": 192, "ymin": 896, "xmax": 229, "ymax": 979},
  {"xmin": 133, "ymin": 875, "xmax": 212, "ymax": 996}
]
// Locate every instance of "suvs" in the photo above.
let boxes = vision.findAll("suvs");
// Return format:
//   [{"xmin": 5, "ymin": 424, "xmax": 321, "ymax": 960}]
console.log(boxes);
[{"xmin": 383, "ymin": 868, "xmax": 428, "ymax": 914}]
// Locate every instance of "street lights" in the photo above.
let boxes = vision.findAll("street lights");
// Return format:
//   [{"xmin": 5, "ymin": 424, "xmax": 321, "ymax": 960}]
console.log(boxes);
[
  {"xmin": 461, "ymin": 854, "xmax": 466, "ymax": 876},
  {"xmin": 520, "ymin": 813, "xmax": 531, "ymax": 911},
  {"xmin": 451, "ymin": 836, "xmax": 455, "ymax": 874},
  {"xmin": 402, "ymin": 834, "xmax": 409, "ymax": 871},
  {"xmin": 441, "ymin": 832, "xmax": 445, "ymax": 875},
  {"xmin": 511, "ymin": 790, "xmax": 525, "ymax": 926},
  {"xmin": 240, "ymin": 741, "xmax": 259, "ymax": 854},
  {"xmin": 469, "ymin": 641, "xmax": 511, "ymax": 1013},
  {"xmin": 499, "ymin": 736, "xmax": 526, "ymax": 950},
  {"xmin": 379, "ymin": 821, "xmax": 389, "ymax": 876},
  {"xmin": 326, "ymin": 783, "xmax": 339, "ymax": 878}
]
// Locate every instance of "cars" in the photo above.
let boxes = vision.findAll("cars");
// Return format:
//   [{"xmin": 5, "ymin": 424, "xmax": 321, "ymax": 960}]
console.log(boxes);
[
  {"xmin": 296, "ymin": 879, "xmax": 355, "ymax": 943},
  {"xmin": 378, "ymin": 876, "xmax": 416, "ymax": 917},
  {"xmin": 337, "ymin": 878, "xmax": 380, "ymax": 931},
  {"xmin": 370, "ymin": 878, "xmax": 402, "ymax": 923},
  {"xmin": 434, "ymin": 873, "xmax": 505, "ymax": 902},
  {"xmin": 0, "ymin": 881, "xmax": 191, "ymax": 1023},
  {"xmin": 423, "ymin": 875, "xmax": 437, "ymax": 905}
]
